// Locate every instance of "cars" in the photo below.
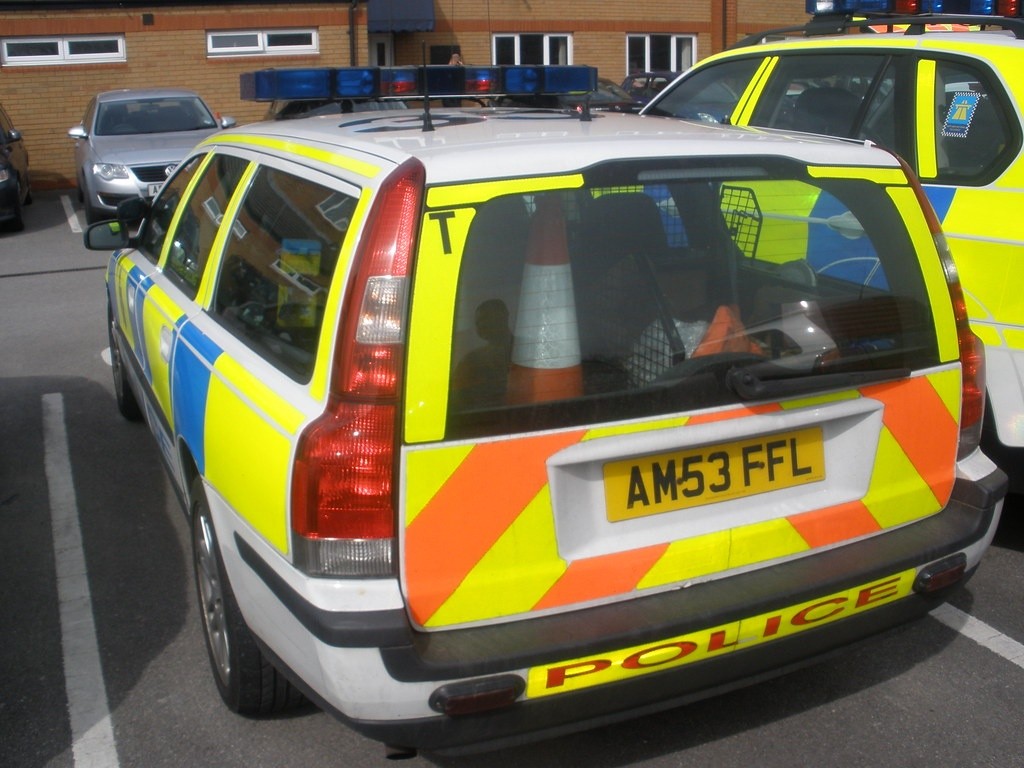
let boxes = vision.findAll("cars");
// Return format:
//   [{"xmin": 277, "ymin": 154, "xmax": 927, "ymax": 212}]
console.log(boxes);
[
  {"xmin": 620, "ymin": 71, "xmax": 740, "ymax": 123},
  {"xmin": 0, "ymin": 102, "xmax": 32, "ymax": 232},
  {"xmin": 487, "ymin": 77, "xmax": 646, "ymax": 114},
  {"xmin": 264, "ymin": 96, "xmax": 407, "ymax": 121},
  {"xmin": 68, "ymin": 88, "xmax": 236, "ymax": 224}
]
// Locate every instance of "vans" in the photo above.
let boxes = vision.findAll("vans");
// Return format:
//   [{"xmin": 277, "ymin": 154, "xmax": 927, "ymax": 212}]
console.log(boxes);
[{"xmin": 84, "ymin": 91, "xmax": 1011, "ymax": 749}]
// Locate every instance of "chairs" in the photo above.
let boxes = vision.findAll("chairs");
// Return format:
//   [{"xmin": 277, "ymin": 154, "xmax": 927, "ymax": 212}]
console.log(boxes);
[
  {"xmin": 161, "ymin": 106, "xmax": 191, "ymax": 129},
  {"xmin": 100, "ymin": 104, "xmax": 138, "ymax": 134},
  {"xmin": 574, "ymin": 192, "xmax": 669, "ymax": 290},
  {"xmin": 773, "ymin": 87, "xmax": 864, "ymax": 137}
]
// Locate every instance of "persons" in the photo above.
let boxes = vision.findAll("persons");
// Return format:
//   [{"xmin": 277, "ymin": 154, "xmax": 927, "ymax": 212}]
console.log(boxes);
[
  {"xmin": 450, "ymin": 299, "xmax": 514, "ymax": 411},
  {"xmin": 441, "ymin": 53, "xmax": 465, "ymax": 108}
]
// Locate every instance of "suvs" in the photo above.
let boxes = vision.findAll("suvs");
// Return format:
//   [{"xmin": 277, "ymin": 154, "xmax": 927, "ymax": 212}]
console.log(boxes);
[{"xmin": 591, "ymin": 10, "xmax": 1024, "ymax": 496}]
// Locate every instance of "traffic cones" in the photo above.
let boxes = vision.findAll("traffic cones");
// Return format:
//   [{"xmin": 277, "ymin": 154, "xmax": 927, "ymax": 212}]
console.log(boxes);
[{"xmin": 503, "ymin": 194, "xmax": 582, "ymax": 403}]
